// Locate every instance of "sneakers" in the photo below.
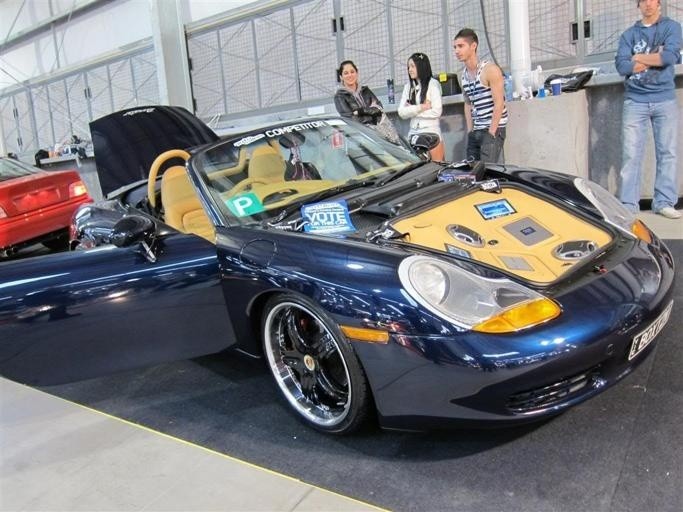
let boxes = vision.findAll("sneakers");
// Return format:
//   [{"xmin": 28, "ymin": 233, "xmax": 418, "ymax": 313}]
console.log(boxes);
[{"xmin": 659, "ymin": 205, "xmax": 681, "ymax": 219}]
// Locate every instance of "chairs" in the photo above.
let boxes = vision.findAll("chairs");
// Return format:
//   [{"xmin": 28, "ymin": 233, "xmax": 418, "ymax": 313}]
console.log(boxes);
[
  {"xmin": 248, "ymin": 145, "xmax": 286, "ymax": 193},
  {"xmin": 160, "ymin": 165, "xmax": 217, "ymax": 246}
]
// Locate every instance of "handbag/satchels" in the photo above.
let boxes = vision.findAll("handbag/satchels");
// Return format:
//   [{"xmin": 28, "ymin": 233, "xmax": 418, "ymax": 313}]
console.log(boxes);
[{"xmin": 366, "ymin": 113, "xmax": 400, "ymax": 145}]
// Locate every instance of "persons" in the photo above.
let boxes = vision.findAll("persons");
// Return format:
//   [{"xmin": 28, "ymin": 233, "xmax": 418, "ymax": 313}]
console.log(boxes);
[
  {"xmin": 333, "ymin": 60, "xmax": 383, "ymax": 131},
  {"xmin": 397, "ymin": 53, "xmax": 446, "ymax": 162},
  {"xmin": 453, "ymin": 27, "xmax": 508, "ymax": 163},
  {"xmin": 615, "ymin": 0, "xmax": 683, "ymax": 219}
]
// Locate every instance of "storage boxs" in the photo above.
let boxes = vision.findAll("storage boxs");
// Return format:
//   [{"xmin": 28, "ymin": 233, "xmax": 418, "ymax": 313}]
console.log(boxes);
[{"xmin": 433, "ymin": 73, "xmax": 461, "ymax": 96}]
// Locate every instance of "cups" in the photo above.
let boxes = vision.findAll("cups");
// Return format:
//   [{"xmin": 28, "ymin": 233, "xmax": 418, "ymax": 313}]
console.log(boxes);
[
  {"xmin": 62, "ymin": 146, "xmax": 70, "ymax": 155},
  {"xmin": 518, "ymin": 73, "xmax": 533, "ymax": 101},
  {"xmin": 47, "ymin": 147, "xmax": 56, "ymax": 158},
  {"xmin": 549, "ymin": 79, "xmax": 561, "ymax": 95}
]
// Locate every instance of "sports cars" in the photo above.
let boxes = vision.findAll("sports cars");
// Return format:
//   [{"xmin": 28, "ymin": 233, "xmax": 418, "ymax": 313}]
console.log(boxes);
[{"xmin": 0, "ymin": 105, "xmax": 676, "ymax": 444}]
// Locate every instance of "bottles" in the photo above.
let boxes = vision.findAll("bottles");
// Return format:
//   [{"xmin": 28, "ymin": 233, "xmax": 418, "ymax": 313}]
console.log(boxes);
[
  {"xmin": 537, "ymin": 70, "xmax": 546, "ymax": 98},
  {"xmin": 386, "ymin": 78, "xmax": 395, "ymax": 103},
  {"xmin": 502, "ymin": 72, "xmax": 513, "ymax": 102}
]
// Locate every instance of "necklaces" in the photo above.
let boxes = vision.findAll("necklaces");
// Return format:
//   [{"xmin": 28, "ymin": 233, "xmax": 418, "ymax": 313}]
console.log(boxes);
[{"xmin": 462, "ymin": 65, "xmax": 479, "ymax": 119}]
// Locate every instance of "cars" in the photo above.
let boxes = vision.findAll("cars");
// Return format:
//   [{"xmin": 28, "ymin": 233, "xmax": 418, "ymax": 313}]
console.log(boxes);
[{"xmin": 0, "ymin": 154, "xmax": 93, "ymax": 261}]
